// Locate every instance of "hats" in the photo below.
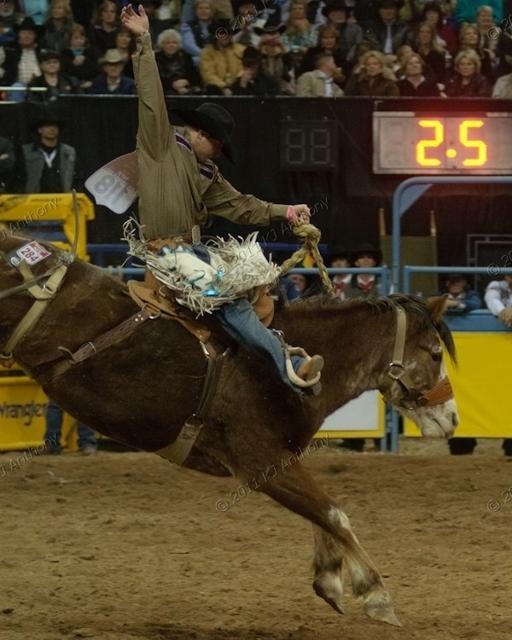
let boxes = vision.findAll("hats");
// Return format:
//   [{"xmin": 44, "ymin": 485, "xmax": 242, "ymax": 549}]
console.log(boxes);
[
  {"xmin": 176, "ymin": 103, "xmax": 238, "ymax": 164},
  {"xmin": 2, "ymin": 3, "xmax": 410, "ymax": 67},
  {"xmin": 29, "ymin": 112, "xmax": 65, "ymax": 131},
  {"xmin": 437, "ymin": 262, "xmax": 475, "ymax": 283},
  {"xmin": 350, "ymin": 245, "xmax": 385, "ymax": 265}
]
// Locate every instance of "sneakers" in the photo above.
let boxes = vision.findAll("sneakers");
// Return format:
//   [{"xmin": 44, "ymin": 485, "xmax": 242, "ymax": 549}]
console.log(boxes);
[
  {"xmin": 297, "ymin": 355, "xmax": 325, "ymax": 398},
  {"xmin": 29, "ymin": 444, "xmax": 61, "ymax": 456},
  {"xmin": 77, "ymin": 439, "xmax": 100, "ymax": 456}
]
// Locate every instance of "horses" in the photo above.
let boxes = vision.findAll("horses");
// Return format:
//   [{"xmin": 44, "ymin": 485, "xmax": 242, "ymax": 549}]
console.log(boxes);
[{"xmin": 0, "ymin": 225, "xmax": 460, "ymax": 628}]
